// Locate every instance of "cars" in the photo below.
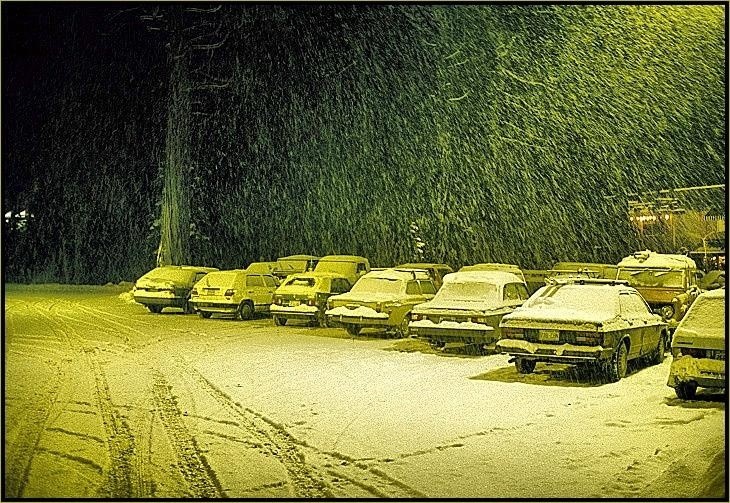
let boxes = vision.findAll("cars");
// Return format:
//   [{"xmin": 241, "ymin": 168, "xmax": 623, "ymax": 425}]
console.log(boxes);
[
  {"xmin": 407, "ymin": 271, "xmax": 533, "ymax": 352},
  {"xmin": 324, "ymin": 271, "xmax": 441, "ymax": 336},
  {"xmin": 134, "ymin": 265, "xmax": 219, "ymax": 315},
  {"xmin": 495, "ymin": 278, "xmax": 668, "ymax": 381},
  {"xmin": 270, "ymin": 273, "xmax": 354, "ymax": 329},
  {"xmin": 249, "ymin": 254, "xmax": 386, "ymax": 277},
  {"xmin": 396, "ymin": 262, "xmax": 454, "ymax": 284},
  {"xmin": 459, "ymin": 253, "xmax": 712, "ymax": 318},
  {"xmin": 189, "ymin": 270, "xmax": 284, "ymax": 322}
]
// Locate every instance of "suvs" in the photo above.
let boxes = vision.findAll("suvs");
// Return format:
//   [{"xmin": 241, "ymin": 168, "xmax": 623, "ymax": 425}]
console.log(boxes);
[{"xmin": 665, "ymin": 289, "xmax": 726, "ymax": 400}]
